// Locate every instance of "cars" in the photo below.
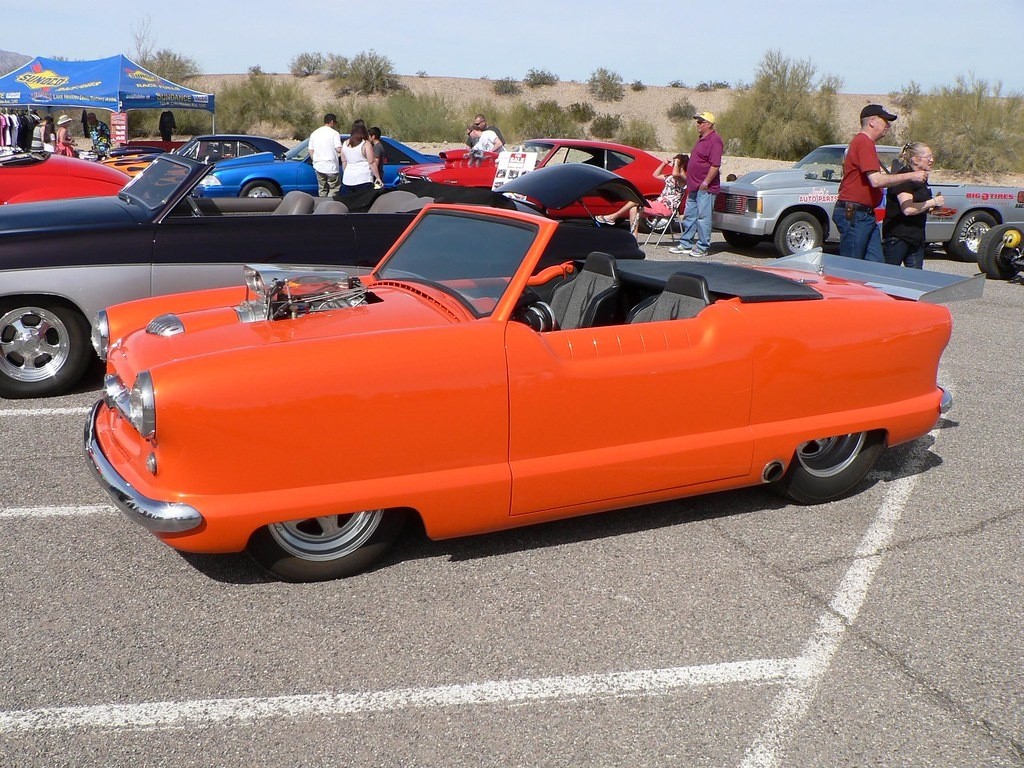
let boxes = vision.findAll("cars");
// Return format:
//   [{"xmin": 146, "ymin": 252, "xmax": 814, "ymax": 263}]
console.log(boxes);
[
  {"xmin": 188, "ymin": 134, "xmax": 445, "ymax": 198},
  {"xmin": 0, "ymin": 153, "xmax": 652, "ymax": 400},
  {"xmin": 82, "ymin": 203, "xmax": 952, "ymax": 583},
  {"xmin": 98, "ymin": 134, "xmax": 306, "ymax": 186},
  {"xmin": 0, "ymin": 151, "xmax": 134, "ymax": 206},
  {"xmin": 398, "ymin": 139, "xmax": 690, "ymax": 234}
]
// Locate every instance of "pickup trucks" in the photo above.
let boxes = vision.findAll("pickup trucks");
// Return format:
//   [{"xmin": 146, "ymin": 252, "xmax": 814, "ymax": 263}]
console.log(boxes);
[{"xmin": 710, "ymin": 144, "xmax": 1024, "ymax": 263}]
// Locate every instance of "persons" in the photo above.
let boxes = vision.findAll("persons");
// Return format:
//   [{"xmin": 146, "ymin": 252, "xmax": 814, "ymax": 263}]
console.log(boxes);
[
  {"xmin": 883, "ymin": 139, "xmax": 945, "ymax": 270},
  {"xmin": 31, "ymin": 116, "xmax": 53, "ymax": 152},
  {"xmin": 595, "ymin": 154, "xmax": 689, "ymax": 248},
  {"xmin": 831, "ymin": 104, "xmax": 928, "ymax": 262},
  {"xmin": 308, "ymin": 114, "xmax": 341, "ymax": 199},
  {"xmin": 342, "ymin": 119, "xmax": 384, "ymax": 194},
  {"xmin": 466, "ymin": 124, "xmax": 507, "ymax": 152},
  {"xmin": 56, "ymin": 115, "xmax": 78, "ymax": 156},
  {"xmin": 368, "ymin": 127, "xmax": 383, "ymax": 182},
  {"xmin": 466, "ymin": 114, "xmax": 505, "ymax": 148},
  {"xmin": 88, "ymin": 112, "xmax": 110, "ymax": 152},
  {"xmin": 668, "ymin": 112, "xmax": 723, "ymax": 257}
]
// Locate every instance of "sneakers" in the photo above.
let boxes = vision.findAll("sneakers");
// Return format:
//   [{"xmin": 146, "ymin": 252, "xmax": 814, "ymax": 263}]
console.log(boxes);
[
  {"xmin": 689, "ymin": 248, "xmax": 709, "ymax": 258},
  {"xmin": 668, "ymin": 243, "xmax": 693, "ymax": 254}
]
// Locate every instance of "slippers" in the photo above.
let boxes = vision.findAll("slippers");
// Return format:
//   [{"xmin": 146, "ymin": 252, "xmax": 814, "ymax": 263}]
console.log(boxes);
[{"xmin": 595, "ymin": 215, "xmax": 615, "ymax": 225}]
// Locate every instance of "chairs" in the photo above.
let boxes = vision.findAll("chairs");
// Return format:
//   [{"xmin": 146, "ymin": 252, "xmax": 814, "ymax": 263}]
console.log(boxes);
[
  {"xmin": 645, "ymin": 192, "xmax": 685, "ymax": 248},
  {"xmin": 549, "ymin": 250, "xmax": 621, "ymax": 330},
  {"xmin": 271, "ymin": 190, "xmax": 349, "ymax": 214},
  {"xmin": 625, "ymin": 271, "xmax": 712, "ymax": 324}
]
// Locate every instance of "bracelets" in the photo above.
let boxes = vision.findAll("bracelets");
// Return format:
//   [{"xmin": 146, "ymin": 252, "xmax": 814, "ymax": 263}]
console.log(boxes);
[
  {"xmin": 702, "ymin": 181, "xmax": 708, "ymax": 186},
  {"xmin": 932, "ymin": 198, "xmax": 937, "ymax": 206}
]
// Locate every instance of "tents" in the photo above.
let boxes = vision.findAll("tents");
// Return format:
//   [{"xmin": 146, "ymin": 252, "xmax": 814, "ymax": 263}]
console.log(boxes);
[{"xmin": 0, "ymin": 53, "xmax": 215, "ymax": 136}]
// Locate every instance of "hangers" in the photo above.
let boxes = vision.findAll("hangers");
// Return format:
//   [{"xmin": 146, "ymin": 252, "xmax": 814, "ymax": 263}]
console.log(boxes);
[{"xmin": 0, "ymin": 107, "xmax": 36, "ymax": 116}]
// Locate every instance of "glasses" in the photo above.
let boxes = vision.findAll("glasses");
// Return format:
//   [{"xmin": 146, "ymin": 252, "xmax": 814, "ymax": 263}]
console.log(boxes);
[
  {"xmin": 917, "ymin": 155, "xmax": 933, "ymax": 159},
  {"xmin": 334, "ymin": 121, "xmax": 337, "ymax": 124},
  {"xmin": 697, "ymin": 121, "xmax": 707, "ymax": 124},
  {"xmin": 467, "ymin": 130, "xmax": 472, "ymax": 136},
  {"xmin": 476, "ymin": 121, "xmax": 483, "ymax": 125},
  {"xmin": 88, "ymin": 117, "xmax": 95, "ymax": 119}
]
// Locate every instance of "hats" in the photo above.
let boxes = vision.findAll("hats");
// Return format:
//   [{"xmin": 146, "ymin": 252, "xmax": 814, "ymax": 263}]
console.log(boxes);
[
  {"xmin": 860, "ymin": 105, "xmax": 897, "ymax": 121},
  {"xmin": 57, "ymin": 115, "xmax": 72, "ymax": 125},
  {"xmin": 693, "ymin": 111, "xmax": 716, "ymax": 124}
]
